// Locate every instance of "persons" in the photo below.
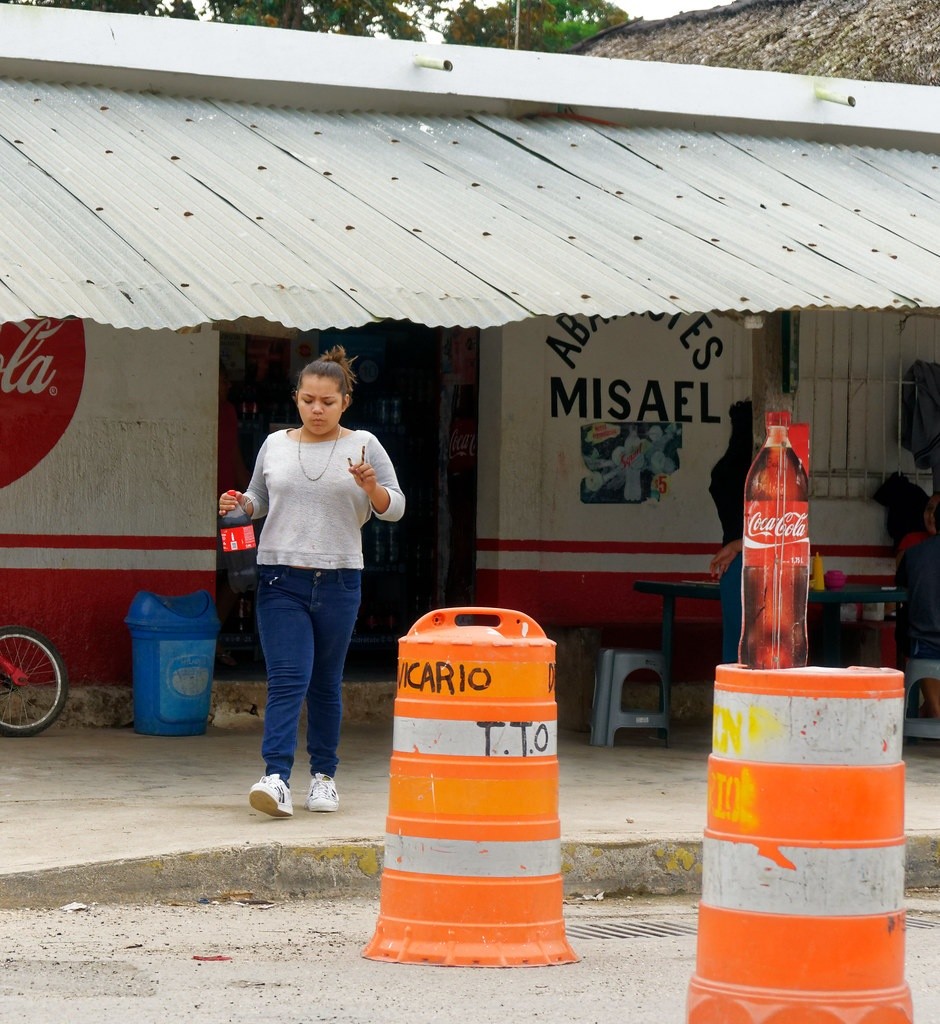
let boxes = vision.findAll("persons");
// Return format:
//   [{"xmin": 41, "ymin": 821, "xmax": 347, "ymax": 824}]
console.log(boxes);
[
  {"xmin": 219, "ymin": 345, "xmax": 405, "ymax": 817},
  {"xmin": 710, "ymin": 399, "xmax": 754, "ymax": 664},
  {"xmin": 895, "ymin": 491, "xmax": 940, "ymax": 718}
]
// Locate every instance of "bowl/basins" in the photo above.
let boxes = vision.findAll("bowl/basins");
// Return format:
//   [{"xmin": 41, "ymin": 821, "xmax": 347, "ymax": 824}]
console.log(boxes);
[{"xmin": 824, "ymin": 570, "xmax": 847, "ymax": 591}]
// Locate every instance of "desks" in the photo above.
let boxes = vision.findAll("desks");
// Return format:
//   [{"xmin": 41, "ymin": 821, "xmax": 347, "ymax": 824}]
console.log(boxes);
[{"xmin": 633, "ymin": 580, "xmax": 908, "ymax": 741}]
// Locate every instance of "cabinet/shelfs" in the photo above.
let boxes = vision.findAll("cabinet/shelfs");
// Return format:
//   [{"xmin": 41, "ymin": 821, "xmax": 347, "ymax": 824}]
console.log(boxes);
[{"xmin": 219, "ymin": 418, "xmax": 409, "ymax": 660}]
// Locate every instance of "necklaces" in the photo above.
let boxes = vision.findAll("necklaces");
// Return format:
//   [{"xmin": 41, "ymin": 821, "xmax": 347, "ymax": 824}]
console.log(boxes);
[{"xmin": 299, "ymin": 425, "xmax": 340, "ymax": 481}]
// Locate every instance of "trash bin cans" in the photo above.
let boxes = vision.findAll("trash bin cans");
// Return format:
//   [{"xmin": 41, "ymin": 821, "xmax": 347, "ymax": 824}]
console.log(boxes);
[{"xmin": 124, "ymin": 587, "xmax": 221, "ymax": 736}]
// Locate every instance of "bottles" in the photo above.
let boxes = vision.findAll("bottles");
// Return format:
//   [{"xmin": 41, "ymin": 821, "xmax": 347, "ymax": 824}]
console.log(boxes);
[
  {"xmin": 219, "ymin": 489, "xmax": 261, "ymax": 594},
  {"xmin": 813, "ymin": 552, "xmax": 824, "ymax": 591},
  {"xmin": 739, "ymin": 410, "xmax": 809, "ymax": 668}
]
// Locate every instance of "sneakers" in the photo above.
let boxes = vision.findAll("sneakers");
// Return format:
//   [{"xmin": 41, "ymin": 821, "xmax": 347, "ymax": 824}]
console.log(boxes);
[
  {"xmin": 248, "ymin": 773, "xmax": 294, "ymax": 818},
  {"xmin": 303, "ymin": 772, "xmax": 340, "ymax": 812}
]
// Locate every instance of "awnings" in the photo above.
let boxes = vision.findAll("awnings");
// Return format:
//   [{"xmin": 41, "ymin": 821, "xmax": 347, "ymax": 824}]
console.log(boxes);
[{"xmin": 0, "ymin": 76, "xmax": 940, "ymax": 331}]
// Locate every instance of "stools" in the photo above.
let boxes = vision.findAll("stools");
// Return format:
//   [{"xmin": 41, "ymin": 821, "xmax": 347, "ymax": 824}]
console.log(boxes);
[
  {"xmin": 903, "ymin": 659, "xmax": 940, "ymax": 739},
  {"xmin": 585, "ymin": 649, "xmax": 672, "ymax": 749}
]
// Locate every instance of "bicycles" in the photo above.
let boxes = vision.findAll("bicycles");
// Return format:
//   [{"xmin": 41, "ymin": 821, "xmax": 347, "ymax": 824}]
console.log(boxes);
[{"xmin": 0, "ymin": 626, "xmax": 68, "ymax": 737}]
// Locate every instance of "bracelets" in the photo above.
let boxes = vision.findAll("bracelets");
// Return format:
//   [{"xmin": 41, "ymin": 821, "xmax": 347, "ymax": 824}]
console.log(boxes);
[{"xmin": 243, "ymin": 497, "xmax": 255, "ymax": 509}]
